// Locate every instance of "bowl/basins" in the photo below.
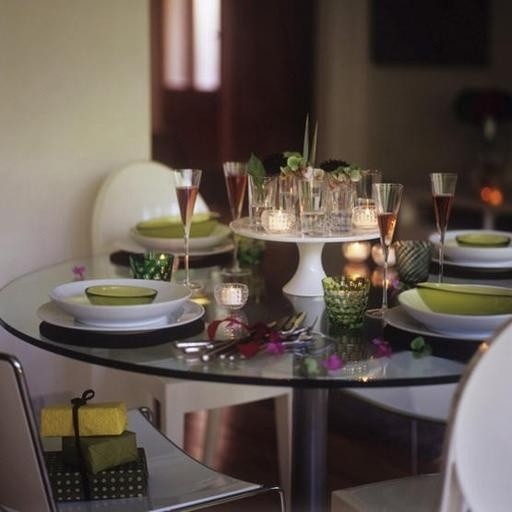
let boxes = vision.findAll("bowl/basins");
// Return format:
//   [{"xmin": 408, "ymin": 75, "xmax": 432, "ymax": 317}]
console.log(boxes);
[
  {"xmin": 397, "ymin": 285, "xmax": 511, "ymax": 336},
  {"xmin": 86, "ymin": 286, "xmax": 159, "ymax": 304},
  {"xmin": 49, "ymin": 278, "xmax": 193, "ymax": 327},
  {"xmin": 418, "ymin": 281, "xmax": 509, "ymax": 313},
  {"xmin": 429, "ymin": 231, "xmax": 512, "ymax": 263},
  {"xmin": 454, "ymin": 233, "xmax": 510, "ymax": 246}
]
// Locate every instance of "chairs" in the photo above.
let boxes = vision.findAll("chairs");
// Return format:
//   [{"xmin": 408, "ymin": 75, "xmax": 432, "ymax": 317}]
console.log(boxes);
[
  {"xmin": 0, "ymin": 355, "xmax": 288, "ymax": 511},
  {"xmin": 89, "ymin": 160, "xmax": 294, "ymax": 511},
  {"xmin": 331, "ymin": 318, "xmax": 512, "ymax": 511},
  {"xmin": 330, "ymin": 350, "xmax": 467, "ymax": 477}
]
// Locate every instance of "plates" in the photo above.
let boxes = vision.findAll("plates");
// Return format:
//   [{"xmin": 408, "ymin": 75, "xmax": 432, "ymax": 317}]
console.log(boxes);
[
  {"xmin": 115, "ymin": 216, "xmax": 235, "ymax": 262},
  {"xmin": 431, "ymin": 256, "xmax": 511, "ymax": 271},
  {"xmin": 37, "ymin": 299, "xmax": 206, "ymax": 336},
  {"xmin": 385, "ymin": 305, "xmax": 494, "ymax": 343}
]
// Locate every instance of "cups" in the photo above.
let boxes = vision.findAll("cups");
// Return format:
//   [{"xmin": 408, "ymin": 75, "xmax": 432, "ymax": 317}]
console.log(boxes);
[
  {"xmin": 243, "ymin": 173, "xmax": 381, "ymax": 237},
  {"xmin": 216, "ymin": 282, "xmax": 248, "ymax": 311},
  {"xmin": 341, "ymin": 262, "xmax": 400, "ymax": 291},
  {"xmin": 395, "ymin": 239, "xmax": 433, "ymax": 287},
  {"xmin": 129, "ymin": 252, "xmax": 174, "ymax": 279},
  {"xmin": 321, "ymin": 276, "xmax": 369, "ymax": 327},
  {"xmin": 341, "ymin": 242, "xmax": 395, "ymax": 268}
]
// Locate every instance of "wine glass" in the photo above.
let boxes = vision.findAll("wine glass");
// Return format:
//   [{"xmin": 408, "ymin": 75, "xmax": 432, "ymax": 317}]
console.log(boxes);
[
  {"xmin": 222, "ymin": 161, "xmax": 249, "ymax": 280},
  {"xmin": 370, "ymin": 182, "xmax": 404, "ymax": 320},
  {"xmin": 174, "ymin": 169, "xmax": 204, "ymax": 292},
  {"xmin": 430, "ymin": 172, "xmax": 457, "ymax": 282}
]
